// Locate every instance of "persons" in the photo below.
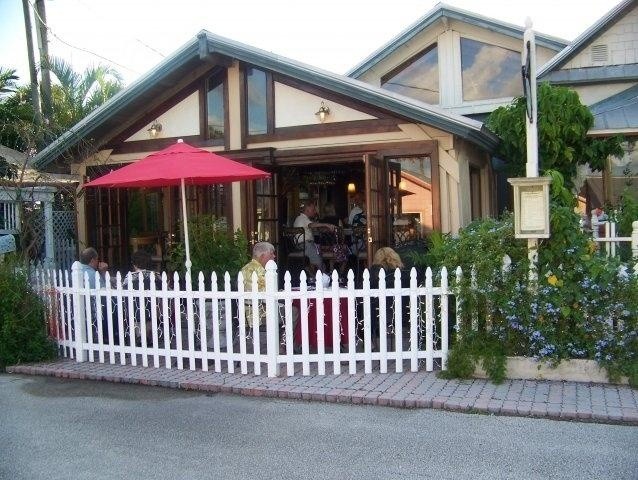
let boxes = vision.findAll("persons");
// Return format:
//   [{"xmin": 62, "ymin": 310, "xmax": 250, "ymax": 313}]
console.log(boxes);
[
  {"xmin": 373, "ymin": 247, "xmax": 404, "ymax": 273},
  {"xmin": 293, "ymin": 193, "xmax": 366, "ymax": 269},
  {"xmin": 591, "ymin": 208, "xmax": 608, "ymax": 256},
  {"xmin": 242, "ymin": 242, "xmax": 299, "ymax": 351},
  {"xmin": 82, "ymin": 248, "xmax": 173, "ymax": 346}
]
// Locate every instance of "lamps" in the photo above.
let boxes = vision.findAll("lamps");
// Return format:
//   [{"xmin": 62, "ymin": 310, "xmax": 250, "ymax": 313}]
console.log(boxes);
[
  {"xmin": 314, "ymin": 100, "xmax": 330, "ymax": 120},
  {"xmin": 147, "ymin": 118, "xmax": 163, "ymax": 136}
]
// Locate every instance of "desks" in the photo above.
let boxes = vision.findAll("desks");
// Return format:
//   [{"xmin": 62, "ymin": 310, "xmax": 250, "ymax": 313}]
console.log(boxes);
[
  {"xmin": 290, "ymin": 285, "xmax": 349, "ymax": 352},
  {"xmin": 130, "ymin": 233, "xmax": 163, "ymax": 273}
]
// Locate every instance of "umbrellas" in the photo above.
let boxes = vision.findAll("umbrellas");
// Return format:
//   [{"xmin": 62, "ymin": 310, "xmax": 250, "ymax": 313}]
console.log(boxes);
[{"xmin": 80, "ymin": 139, "xmax": 272, "ymax": 275}]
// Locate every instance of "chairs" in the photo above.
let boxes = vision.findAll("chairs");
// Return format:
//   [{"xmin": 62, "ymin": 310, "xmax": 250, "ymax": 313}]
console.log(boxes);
[
  {"xmin": 222, "ymin": 273, "xmax": 284, "ymax": 368},
  {"xmin": 281, "ymin": 224, "xmax": 368, "ymax": 280},
  {"xmin": 121, "ymin": 277, "xmax": 173, "ymax": 357}
]
